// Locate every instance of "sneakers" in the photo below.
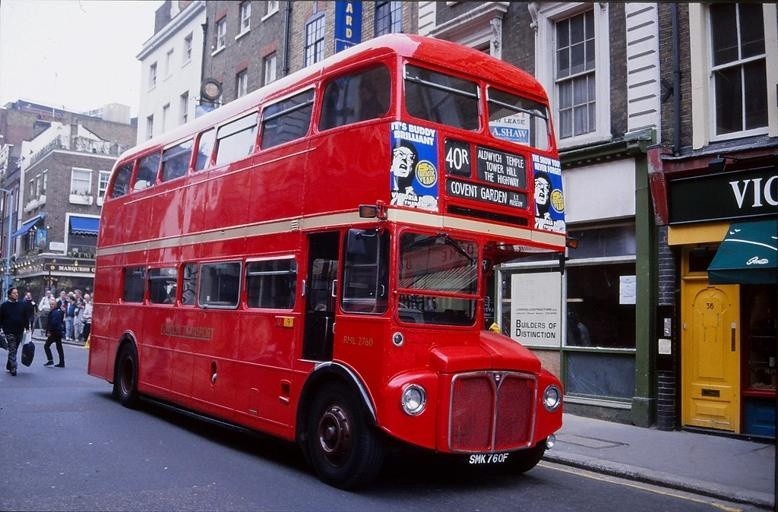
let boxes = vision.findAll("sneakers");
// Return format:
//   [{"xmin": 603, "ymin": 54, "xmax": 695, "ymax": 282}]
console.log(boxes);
[{"xmin": 66, "ymin": 337, "xmax": 80, "ymax": 342}]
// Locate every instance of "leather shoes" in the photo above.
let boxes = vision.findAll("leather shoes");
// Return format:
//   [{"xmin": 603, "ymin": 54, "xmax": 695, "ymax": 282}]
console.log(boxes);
[
  {"xmin": 6, "ymin": 366, "xmax": 16, "ymax": 376},
  {"xmin": 44, "ymin": 361, "xmax": 64, "ymax": 367}
]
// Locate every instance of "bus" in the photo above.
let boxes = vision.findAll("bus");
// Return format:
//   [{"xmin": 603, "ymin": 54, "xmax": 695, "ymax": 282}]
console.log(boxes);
[{"xmin": 85, "ymin": 31, "xmax": 573, "ymax": 488}]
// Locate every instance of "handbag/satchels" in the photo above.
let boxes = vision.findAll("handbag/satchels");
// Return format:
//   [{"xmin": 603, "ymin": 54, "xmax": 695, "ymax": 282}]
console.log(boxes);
[
  {"xmin": 22, "ymin": 342, "xmax": 35, "ymax": 366},
  {"xmin": 0, "ymin": 335, "xmax": 7, "ymax": 350}
]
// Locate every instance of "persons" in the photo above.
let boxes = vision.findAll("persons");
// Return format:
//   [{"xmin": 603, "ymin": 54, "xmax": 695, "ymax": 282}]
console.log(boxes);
[
  {"xmin": 389, "ymin": 140, "xmax": 418, "ymax": 196},
  {"xmin": 23, "ymin": 292, "xmax": 39, "ymax": 335},
  {"xmin": 42, "ymin": 299, "xmax": 66, "ymax": 367},
  {"xmin": 37, "ymin": 289, "xmax": 94, "ymax": 343},
  {"xmin": 0, "ymin": 287, "xmax": 30, "ymax": 376},
  {"xmin": 532, "ymin": 170, "xmax": 553, "ymax": 220}
]
